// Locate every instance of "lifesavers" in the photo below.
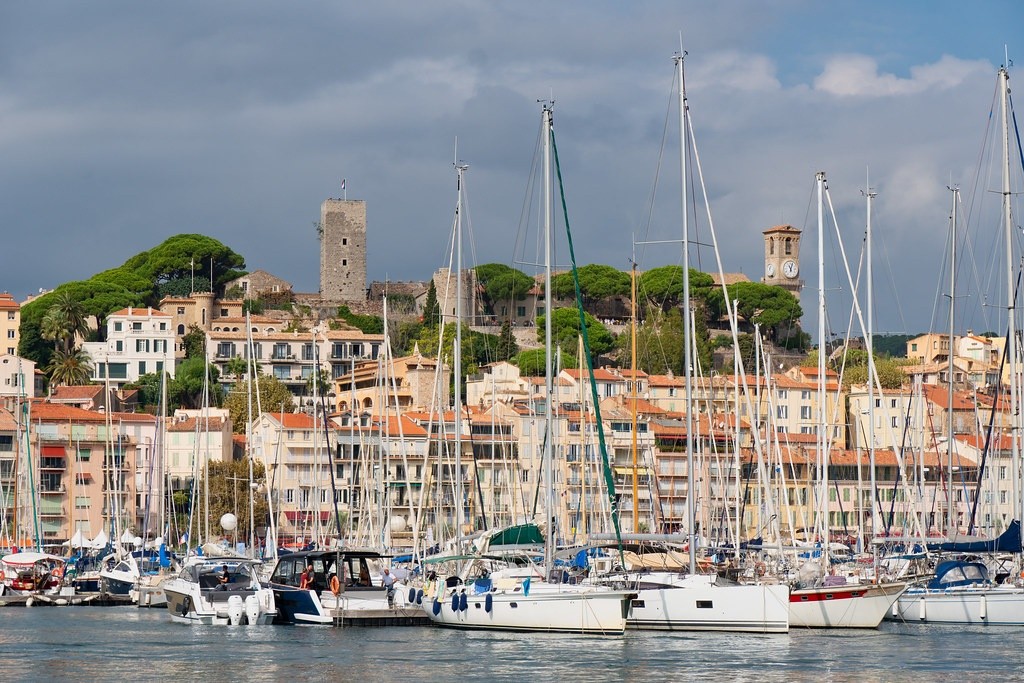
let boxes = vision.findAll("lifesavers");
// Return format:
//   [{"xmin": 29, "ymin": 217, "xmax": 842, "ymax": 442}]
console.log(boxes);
[
  {"xmin": 330, "ymin": 575, "xmax": 341, "ymax": 595},
  {"xmin": 51, "ymin": 568, "xmax": 60, "ymax": 576}
]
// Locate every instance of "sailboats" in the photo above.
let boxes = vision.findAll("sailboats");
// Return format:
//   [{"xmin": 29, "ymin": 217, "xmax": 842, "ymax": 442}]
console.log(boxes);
[{"xmin": 0, "ymin": 30, "xmax": 1024, "ymax": 633}]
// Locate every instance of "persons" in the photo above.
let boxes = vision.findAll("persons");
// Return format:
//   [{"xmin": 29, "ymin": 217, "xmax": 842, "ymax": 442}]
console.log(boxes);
[
  {"xmin": 299, "ymin": 565, "xmax": 371, "ymax": 590},
  {"xmin": 216, "ymin": 566, "xmax": 230, "ymax": 583},
  {"xmin": 381, "ymin": 569, "xmax": 396, "ymax": 602}
]
